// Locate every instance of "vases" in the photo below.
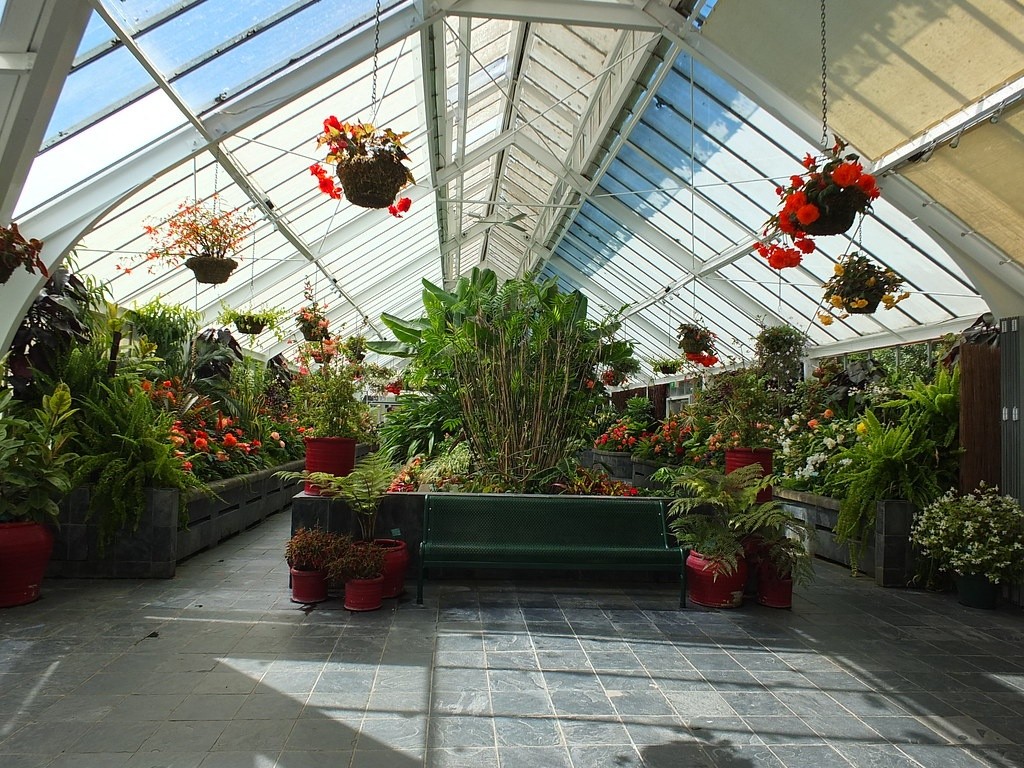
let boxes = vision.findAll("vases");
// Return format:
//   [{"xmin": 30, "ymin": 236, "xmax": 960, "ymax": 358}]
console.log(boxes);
[
  {"xmin": 661, "ymin": 367, "xmax": 677, "ymax": 374},
  {"xmin": 842, "ymin": 290, "xmax": 880, "ymax": 314},
  {"xmin": 348, "ymin": 354, "xmax": 364, "ymax": 364},
  {"xmin": 607, "ymin": 380, "xmax": 619, "ymax": 385},
  {"xmin": 335, "ymin": 160, "xmax": 407, "ymax": 209},
  {"xmin": 352, "ymin": 538, "xmax": 410, "ymax": 600},
  {"xmin": 313, "ymin": 353, "xmax": 331, "ymax": 364},
  {"xmin": 184, "ymin": 252, "xmax": 236, "ymax": 286},
  {"xmin": 290, "ymin": 565, "xmax": 330, "ymax": 602},
  {"xmin": 300, "ymin": 327, "xmax": 323, "ymax": 342},
  {"xmin": 683, "ymin": 341, "xmax": 700, "ymax": 353},
  {"xmin": 723, "ymin": 447, "xmax": 774, "ymax": 502},
  {"xmin": 344, "ymin": 574, "xmax": 383, "ymax": 610},
  {"xmin": 794, "ymin": 199, "xmax": 851, "ymax": 236},
  {"xmin": 954, "ymin": 567, "xmax": 999, "ymax": 608},
  {"xmin": 236, "ymin": 318, "xmax": 263, "ymax": 334}
]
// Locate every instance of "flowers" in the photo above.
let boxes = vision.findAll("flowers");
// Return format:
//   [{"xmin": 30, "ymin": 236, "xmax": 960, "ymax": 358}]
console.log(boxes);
[{"xmin": 0, "ymin": 116, "xmax": 1023, "ymax": 587}]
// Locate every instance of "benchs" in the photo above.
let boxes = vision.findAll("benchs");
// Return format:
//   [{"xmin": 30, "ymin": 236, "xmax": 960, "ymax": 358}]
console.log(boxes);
[{"xmin": 417, "ymin": 491, "xmax": 689, "ymax": 611}]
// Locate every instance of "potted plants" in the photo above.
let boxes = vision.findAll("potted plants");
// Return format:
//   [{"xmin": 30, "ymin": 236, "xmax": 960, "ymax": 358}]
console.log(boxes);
[
  {"xmin": 756, "ymin": 538, "xmax": 799, "ymax": 608},
  {"xmin": 649, "ymin": 460, "xmax": 772, "ymax": 609},
  {"xmin": 292, "ymin": 341, "xmax": 365, "ymax": 493},
  {"xmin": 0, "ymin": 378, "xmax": 79, "ymax": 609}
]
